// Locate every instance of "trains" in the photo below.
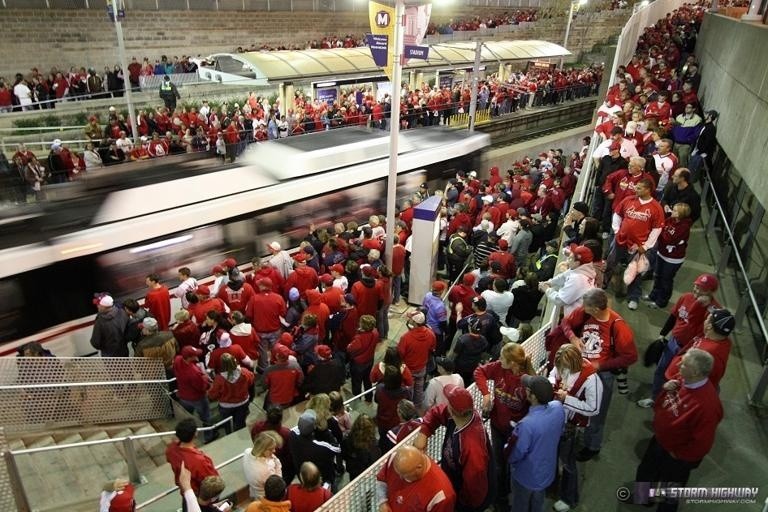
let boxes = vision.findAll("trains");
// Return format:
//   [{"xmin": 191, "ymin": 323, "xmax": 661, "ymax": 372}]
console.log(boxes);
[{"xmin": 0, "ymin": 122, "xmax": 492, "ymax": 398}]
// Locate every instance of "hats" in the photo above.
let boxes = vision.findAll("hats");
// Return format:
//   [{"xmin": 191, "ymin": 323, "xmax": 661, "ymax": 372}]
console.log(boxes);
[
  {"xmin": 432, "ymin": 282, "xmax": 447, "ymax": 294},
  {"xmin": 223, "ymin": 259, "xmax": 236, "ymax": 269},
  {"xmin": 215, "ymin": 329, "xmax": 233, "ymax": 349},
  {"xmin": 179, "ymin": 345, "xmax": 201, "ymax": 358},
  {"xmin": 405, "ymin": 310, "xmax": 430, "ymax": 326},
  {"xmin": 91, "ymin": 293, "xmax": 113, "ymax": 309},
  {"xmin": 691, "ymin": 274, "xmax": 717, "ymax": 296},
  {"xmin": 607, "ymin": 125, "xmax": 624, "ymax": 155},
  {"xmin": 288, "ymin": 253, "xmax": 374, "ymax": 309},
  {"xmin": 481, "ymin": 195, "xmax": 495, "ymax": 206},
  {"xmin": 465, "ymin": 274, "xmax": 476, "ymax": 288},
  {"xmin": 705, "ymin": 306, "xmax": 736, "ymax": 339},
  {"xmin": 496, "ymin": 240, "xmax": 509, "ymax": 252},
  {"xmin": 506, "ymin": 207, "xmax": 519, "ymax": 221},
  {"xmin": 267, "ymin": 242, "xmax": 281, "ymax": 253},
  {"xmin": 443, "ymin": 384, "xmax": 474, "ymax": 417},
  {"xmin": 314, "ymin": 345, "xmax": 334, "ymax": 361},
  {"xmin": 468, "ymin": 169, "xmax": 484, "ymax": 184},
  {"xmin": 212, "ymin": 266, "xmax": 225, "ymax": 276},
  {"xmin": 194, "ymin": 284, "xmax": 209, "ymax": 297},
  {"xmin": 137, "ymin": 317, "xmax": 158, "ymax": 332},
  {"xmin": 297, "ymin": 409, "xmax": 319, "ymax": 435},
  {"xmin": 253, "ymin": 277, "xmax": 278, "ymax": 293},
  {"xmin": 496, "ymin": 325, "xmax": 521, "ymax": 343}
]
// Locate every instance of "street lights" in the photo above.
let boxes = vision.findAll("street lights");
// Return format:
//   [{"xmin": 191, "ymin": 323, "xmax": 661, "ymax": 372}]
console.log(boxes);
[
  {"xmin": 632, "ymin": 1, "xmax": 650, "ymax": 16},
  {"xmin": 559, "ymin": 0, "xmax": 589, "ymax": 70}
]
